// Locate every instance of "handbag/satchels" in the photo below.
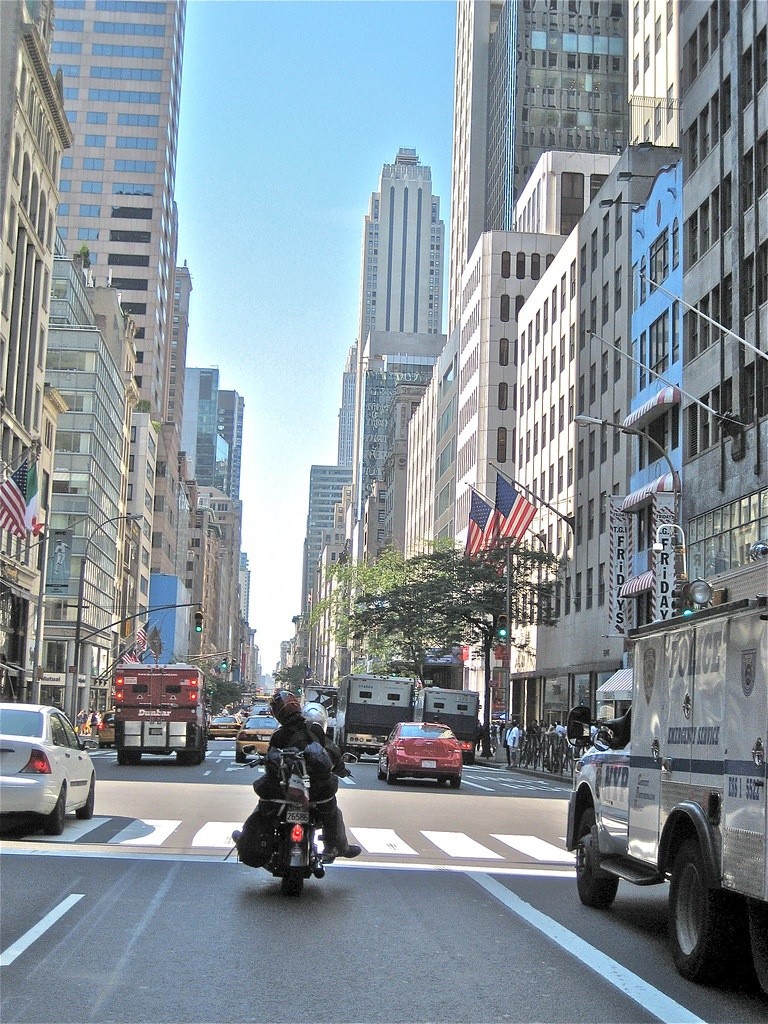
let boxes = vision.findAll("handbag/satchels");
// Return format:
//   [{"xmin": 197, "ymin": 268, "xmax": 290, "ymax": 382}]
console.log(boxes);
[{"xmin": 502, "ymin": 740, "xmax": 508, "ymax": 748}]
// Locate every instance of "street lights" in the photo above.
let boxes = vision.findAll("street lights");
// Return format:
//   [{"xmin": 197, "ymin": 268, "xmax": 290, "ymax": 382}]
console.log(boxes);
[
  {"xmin": 70, "ymin": 513, "xmax": 143, "ymax": 730},
  {"xmin": 574, "ymin": 415, "xmax": 686, "ymax": 590}
]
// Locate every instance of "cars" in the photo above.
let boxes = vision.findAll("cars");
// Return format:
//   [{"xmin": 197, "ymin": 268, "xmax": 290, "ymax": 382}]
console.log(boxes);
[
  {"xmin": 98, "ymin": 710, "xmax": 116, "ymax": 749},
  {"xmin": 235, "ymin": 715, "xmax": 280, "ymax": 763},
  {"xmin": 208, "ymin": 714, "xmax": 249, "ymax": 740},
  {"xmin": 0, "ymin": 703, "xmax": 97, "ymax": 835}
]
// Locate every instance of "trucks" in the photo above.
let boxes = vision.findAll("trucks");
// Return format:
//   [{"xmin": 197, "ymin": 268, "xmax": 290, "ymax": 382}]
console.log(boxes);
[
  {"xmin": 334, "ymin": 674, "xmax": 417, "ymax": 763},
  {"xmin": 301, "ymin": 685, "xmax": 338, "ymax": 740},
  {"xmin": 115, "ymin": 663, "xmax": 211, "ymax": 765},
  {"xmin": 413, "ymin": 685, "xmax": 479, "ymax": 766}
]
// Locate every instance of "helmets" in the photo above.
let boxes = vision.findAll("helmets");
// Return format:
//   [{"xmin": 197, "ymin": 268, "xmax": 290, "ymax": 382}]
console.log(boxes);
[
  {"xmin": 269, "ymin": 689, "xmax": 301, "ymax": 719},
  {"xmin": 300, "ymin": 701, "xmax": 329, "ymax": 732}
]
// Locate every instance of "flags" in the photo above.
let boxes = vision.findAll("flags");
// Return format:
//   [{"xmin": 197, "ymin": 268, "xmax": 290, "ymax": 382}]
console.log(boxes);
[
  {"xmin": 122, "ymin": 643, "xmax": 141, "ymax": 664},
  {"xmin": 136, "ymin": 621, "xmax": 149, "ymax": 652},
  {"xmin": 0, "ymin": 456, "xmax": 45, "ymax": 540},
  {"xmin": 464, "ymin": 472, "xmax": 538, "ymax": 575}
]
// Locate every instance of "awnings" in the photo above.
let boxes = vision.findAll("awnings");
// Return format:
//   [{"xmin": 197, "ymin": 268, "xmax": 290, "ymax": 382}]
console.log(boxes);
[
  {"xmin": 620, "ymin": 570, "xmax": 652, "ymax": 598},
  {"xmin": 596, "ymin": 670, "xmax": 634, "ymax": 700},
  {"xmin": 622, "ymin": 386, "xmax": 680, "ymax": 435},
  {"xmin": 622, "ymin": 471, "xmax": 678, "ymax": 513}
]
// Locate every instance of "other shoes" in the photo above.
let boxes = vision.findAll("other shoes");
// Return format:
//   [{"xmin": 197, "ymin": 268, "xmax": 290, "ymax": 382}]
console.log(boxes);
[
  {"xmin": 322, "ymin": 847, "xmax": 338, "ymax": 861},
  {"xmin": 336, "ymin": 844, "xmax": 361, "ymax": 858},
  {"xmin": 231, "ymin": 829, "xmax": 243, "ymax": 844}
]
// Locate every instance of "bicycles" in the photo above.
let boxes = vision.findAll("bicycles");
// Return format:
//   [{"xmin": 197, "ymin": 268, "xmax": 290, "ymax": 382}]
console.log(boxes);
[{"xmin": 520, "ymin": 740, "xmax": 540, "ymax": 770}]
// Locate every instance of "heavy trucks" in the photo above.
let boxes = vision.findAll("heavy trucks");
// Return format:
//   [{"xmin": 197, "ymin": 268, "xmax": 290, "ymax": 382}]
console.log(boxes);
[{"xmin": 565, "ymin": 595, "xmax": 768, "ymax": 1002}]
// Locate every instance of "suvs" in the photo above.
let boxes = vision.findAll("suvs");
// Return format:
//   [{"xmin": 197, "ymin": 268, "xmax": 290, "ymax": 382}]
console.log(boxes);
[{"xmin": 250, "ymin": 704, "xmax": 273, "ymax": 715}]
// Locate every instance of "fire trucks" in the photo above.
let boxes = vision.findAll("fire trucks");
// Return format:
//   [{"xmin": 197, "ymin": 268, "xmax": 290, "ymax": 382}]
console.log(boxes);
[{"xmin": 377, "ymin": 721, "xmax": 462, "ymax": 789}]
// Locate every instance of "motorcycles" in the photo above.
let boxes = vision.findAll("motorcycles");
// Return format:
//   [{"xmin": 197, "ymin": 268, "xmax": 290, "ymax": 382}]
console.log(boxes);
[{"xmin": 241, "ymin": 745, "xmax": 358, "ymax": 895}]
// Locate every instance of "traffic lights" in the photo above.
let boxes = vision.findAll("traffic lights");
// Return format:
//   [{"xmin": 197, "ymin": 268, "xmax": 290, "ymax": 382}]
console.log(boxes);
[
  {"xmin": 498, "ymin": 615, "xmax": 507, "ymax": 637},
  {"xmin": 221, "ymin": 657, "xmax": 227, "ymax": 671},
  {"xmin": 682, "ymin": 583, "xmax": 694, "ymax": 616},
  {"xmin": 195, "ymin": 612, "xmax": 202, "ymax": 632},
  {"xmin": 672, "ymin": 589, "xmax": 681, "ymax": 617}
]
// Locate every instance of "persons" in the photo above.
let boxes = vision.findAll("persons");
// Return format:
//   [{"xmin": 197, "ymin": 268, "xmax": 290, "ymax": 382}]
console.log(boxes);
[
  {"xmin": 54, "ymin": 539, "xmax": 70, "ymax": 576},
  {"xmin": 232, "ymin": 691, "xmax": 362, "ymax": 858},
  {"xmin": 475, "ymin": 719, "xmax": 566, "ymax": 771}
]
